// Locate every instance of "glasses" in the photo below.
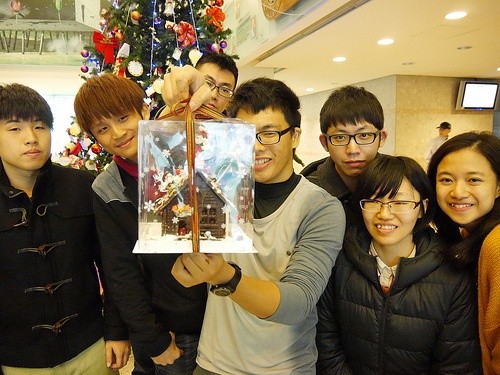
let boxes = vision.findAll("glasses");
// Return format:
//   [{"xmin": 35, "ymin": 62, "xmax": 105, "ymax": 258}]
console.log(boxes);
[
  {"xmin": 205, "ymin": 79, "xmax": 234, "ymax": 99},
  {"xmin": 359, "ymin": 198, "xmax": 422, "ymax": 214},
  {"xmin": 256, "ymin": 126, "xmax": 294, "ymax": 145},
  {"xmin": 324, "ymin": 128, "xmax": 380, "ymax": 146}
]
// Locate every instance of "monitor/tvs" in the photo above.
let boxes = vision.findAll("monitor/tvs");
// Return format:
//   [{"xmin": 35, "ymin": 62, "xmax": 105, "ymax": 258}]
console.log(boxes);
[{"xmin": 455, "ymin": 80, "xmax": 500, "ymax": 110}]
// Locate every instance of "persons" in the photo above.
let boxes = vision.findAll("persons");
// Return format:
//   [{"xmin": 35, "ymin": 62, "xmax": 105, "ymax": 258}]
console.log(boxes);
[
  {"xmin": 150, "ymin": 52, "xmax": 238, "ymax": 121},
  {"xmin": 315, "ymin": 155, "xmax": 479, "ymax": 375},
  {"xmin": 161, "ymin": 65, "xmax": 346, "ymax": 375},
  {"xmin": 74, "ymin": 74, "xmax": 209, "ymax": 375},
  {"xmin": 0, "ymin": 82, "xmax": 131, "ymax": 375},
  {"xmin": 299, "ymin": 85, "xmax": 387, "ymax": 197},
  {"xmin": 426, "ymin": 130, "xmax": 500, "ymax": 375},
  {"xmin": 423, "ymin": 122, "xmax": 451, "ymax": 163}
]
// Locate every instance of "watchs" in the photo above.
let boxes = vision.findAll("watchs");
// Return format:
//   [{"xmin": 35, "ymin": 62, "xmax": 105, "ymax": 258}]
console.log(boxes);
[{"xmin": 210, "ymin": 261, "xmax": 241, "ymax": 296}]
